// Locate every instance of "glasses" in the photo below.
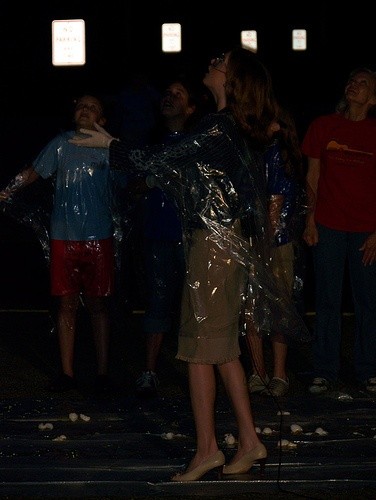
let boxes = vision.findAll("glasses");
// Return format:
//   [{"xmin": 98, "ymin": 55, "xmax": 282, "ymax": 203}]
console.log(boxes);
[{"xmin": 206, "ymin": 59, "xmax": 237, "ymax": 83}]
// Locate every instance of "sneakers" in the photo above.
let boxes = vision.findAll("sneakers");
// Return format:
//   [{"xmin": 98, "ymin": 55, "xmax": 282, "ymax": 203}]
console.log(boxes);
[
  {"xmin": 326, "ymin": 375, "xmax": 376, "ymax": 403},
  {"xmin": 307, "ymin": 372, "xmax": 334, "ymax": 394},
  {"xmin": 266, "ymin": 369, "xmax": 294, "ymax": 394},
  {"xmin": 242, "ymin": 370, "xmax": 264, "ymax": 400},
  {"xmin": 136, "ymin": 372, "xmax": 167, "ymax": 398}
]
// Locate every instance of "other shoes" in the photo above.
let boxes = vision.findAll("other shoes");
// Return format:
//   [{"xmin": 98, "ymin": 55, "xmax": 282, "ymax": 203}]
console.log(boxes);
[
  {"xmin": 48, "ymin": 373, "xmax": 76, "ymax": 394},
  {"xmin": 88, "ymin": 372, "xmax": 108, "ymax": 400}
]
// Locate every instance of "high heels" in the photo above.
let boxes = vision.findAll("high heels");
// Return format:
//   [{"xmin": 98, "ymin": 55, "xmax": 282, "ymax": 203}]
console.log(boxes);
[
  {"xmin": 169, "ymin": 452, "xmax": 224, "ymax": 485},
  {"xmin": 229, "ymin": 436, "xmax": 267, "ymax": 479}
]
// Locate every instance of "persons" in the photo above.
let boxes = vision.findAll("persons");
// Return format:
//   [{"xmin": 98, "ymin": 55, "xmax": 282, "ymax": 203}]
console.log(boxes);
[
  {"xmin": 245, "ymin": 118, "xmax": 299, "ymax": 396},
  {"xmin": 69, "ymin": 49, "xmax": 275, "ymax": 481},
  {"xmin": 135, "ymin": 80, "xmax": 201, "ymax": 388},
  {"xmin": 0, "ymin": 93, "xmax": 130, "ymax": 399},
  {"xmin": 300, "ymin": 69, "xmax": 376, "ymax": 402}
]
